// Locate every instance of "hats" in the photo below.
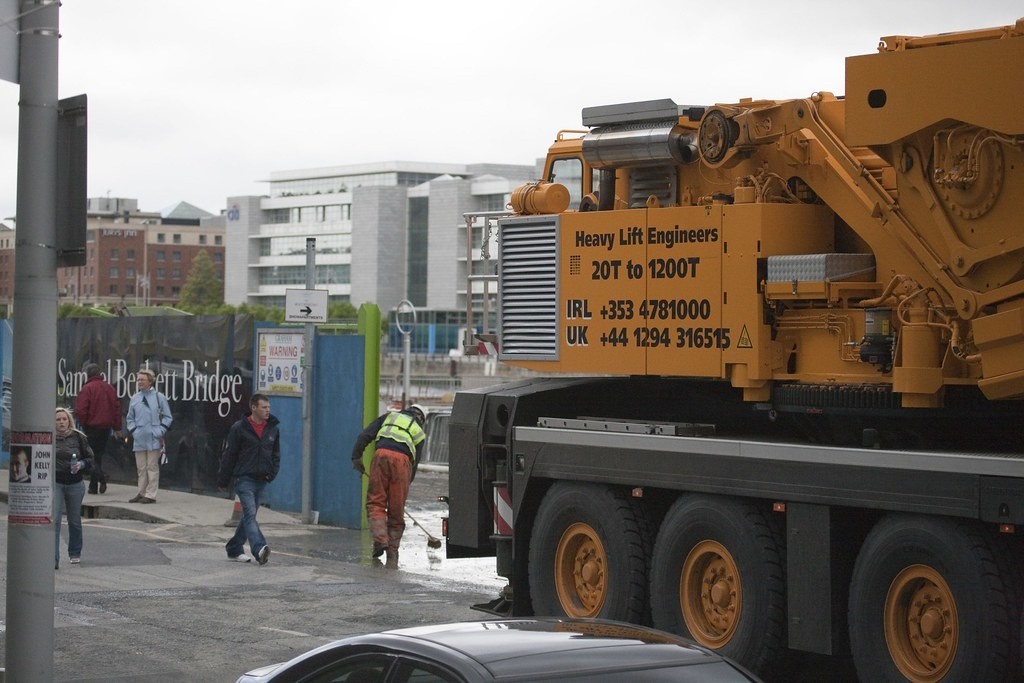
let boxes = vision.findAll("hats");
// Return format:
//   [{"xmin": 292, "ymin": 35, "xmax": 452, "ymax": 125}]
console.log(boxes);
[
  {"xmin": 87, "ymin": 363, "xmax": 101, "ymax": 377},
  {"xmin": 412, "ymin": 403, "xmax": 429, "ymax": 423}
]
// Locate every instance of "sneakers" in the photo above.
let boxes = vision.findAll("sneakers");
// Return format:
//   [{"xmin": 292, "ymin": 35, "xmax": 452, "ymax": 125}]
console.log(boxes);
[
  {"xmin": 258, "ymin": 544, "xmax": 271, "ymax": 565},
  {"xmin": 227, "ymin": 554, "xmax": 251, "ymax": 562}
]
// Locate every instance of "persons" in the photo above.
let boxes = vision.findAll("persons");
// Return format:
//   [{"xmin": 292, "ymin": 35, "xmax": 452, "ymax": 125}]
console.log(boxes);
[
  {"xmin": 217, "ymin": 394, "xmax": 280, "ymax": 564},
  {"xmin": 126, "ymin": 369, "xmax": 173, "ymax": 503},
  {"xmin": 351, "ymin": 404, "xmax": 428, "ymax": 559},
  {"xmin": 55, "ymin": 407, "xmax": 95, "ymax": 569},
  {"xmin": 75, "ymin": 363, "xmax": 122, "ymax": 494},
  {"xmin": 10, "ymin": 447, "xmax": 31, "ymax": 483}
]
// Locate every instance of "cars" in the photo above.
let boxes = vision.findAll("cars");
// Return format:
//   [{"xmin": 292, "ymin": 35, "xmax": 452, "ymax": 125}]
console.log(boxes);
[{"xmin": 235, "ymin": 615, "xmax": 766, "ymax": 682}]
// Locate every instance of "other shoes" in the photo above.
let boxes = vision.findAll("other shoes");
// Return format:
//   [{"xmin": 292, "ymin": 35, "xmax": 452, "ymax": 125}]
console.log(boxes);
[
  {"xmin": 70, "ymin": 555, "xmax": 80, "ymax": 563},
  {"xmin": 88, "ymin": 487, "xmax": 98, "ymax": 494},
  {"xmin": 128, "ymin": 493, "xmax": 143, "ymax": 503},
  {"xmin": 372, "ymin": 542, "xmax": 389, "ymax": 558},
  {"xmin": 98, "ymin": 473, "xmax": 109, "ymax": 493},
  {"xmin": 139, "ymin": 497, "xmax": 156, "ymax": 504}
]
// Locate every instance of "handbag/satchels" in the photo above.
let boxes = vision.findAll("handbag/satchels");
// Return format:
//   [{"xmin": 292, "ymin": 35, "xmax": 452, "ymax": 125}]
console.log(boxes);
[{"xmin": 159, "ymin": 438, "xmax": 169, "ymax": 466}]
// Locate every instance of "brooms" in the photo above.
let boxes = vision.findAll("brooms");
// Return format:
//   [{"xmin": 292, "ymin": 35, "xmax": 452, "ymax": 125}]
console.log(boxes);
[{"xmin": 361, "ymin": 468, "xmax": 441, "ymax": 549}]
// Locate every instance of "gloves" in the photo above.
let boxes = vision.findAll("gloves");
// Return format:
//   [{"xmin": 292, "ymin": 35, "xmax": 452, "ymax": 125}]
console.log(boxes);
[{"xmin": 352, "ymin": 459, "xmax": 365, "ymax": 474}]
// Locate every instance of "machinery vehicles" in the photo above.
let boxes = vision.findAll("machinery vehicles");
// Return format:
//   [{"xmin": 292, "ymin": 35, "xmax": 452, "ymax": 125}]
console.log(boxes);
[{"xmin": 438, "ymin": 16, "xmax": 1024, "ymax": 683}]
